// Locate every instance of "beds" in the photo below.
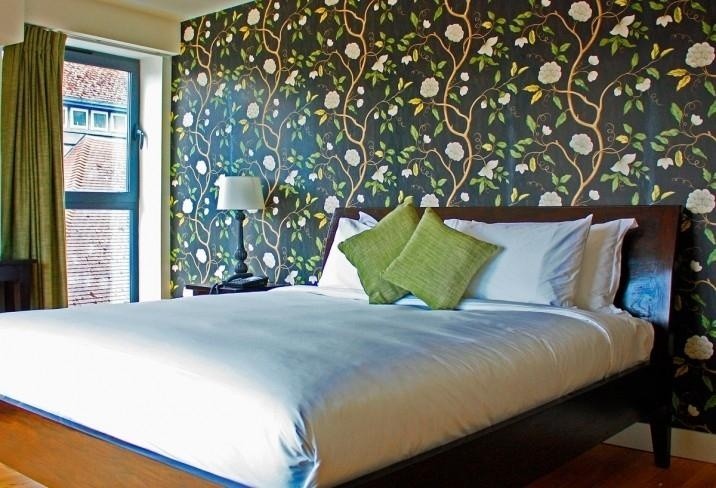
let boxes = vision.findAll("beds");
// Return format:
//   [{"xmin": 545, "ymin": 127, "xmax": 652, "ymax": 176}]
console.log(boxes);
[{"xmin": 1, "ymin": 205, "xmax": 684, "ymax": 488}]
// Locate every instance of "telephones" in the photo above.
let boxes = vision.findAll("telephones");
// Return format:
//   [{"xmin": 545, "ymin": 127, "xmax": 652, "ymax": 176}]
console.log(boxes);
[{"xmin": 222, "ymin": 273, "xmax": 269, "ymax": 288}]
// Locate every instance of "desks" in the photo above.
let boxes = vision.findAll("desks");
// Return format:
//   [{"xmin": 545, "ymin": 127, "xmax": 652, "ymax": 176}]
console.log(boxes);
[{"xmin": 0, "ymin": 259, "xmax": 38, "ymax": 310}]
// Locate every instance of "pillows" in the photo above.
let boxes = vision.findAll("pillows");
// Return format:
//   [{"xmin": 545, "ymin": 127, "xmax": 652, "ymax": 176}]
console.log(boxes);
[
  {"xmin": 443, "ymin": 213, "xmax": 594, "ymax": 309},
  {"xmin": 379, "ymin": 207, "xmax": 505, "ymax": 310},
  {"xmin": 313, "ymin": 211, "xmax": 378, "ymax": 296},
  {"xmin": 574, "ymin": 217, "xmax": 638, "ymax": 314},
  {"xmin": 338, "ymin": 199, "xmax": 421, "ymax": 305}
]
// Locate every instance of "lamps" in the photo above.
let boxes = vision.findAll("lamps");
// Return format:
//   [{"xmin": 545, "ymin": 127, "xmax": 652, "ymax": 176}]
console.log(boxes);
[{"xmin": 216, "ymin": 176, "xmax": 265, "ymax": 273}]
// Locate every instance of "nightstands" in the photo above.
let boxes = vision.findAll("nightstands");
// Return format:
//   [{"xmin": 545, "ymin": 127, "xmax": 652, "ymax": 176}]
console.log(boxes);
[{"xmin": 186, "ymin": 282, "xmax": 290, "ymax": 296}]
